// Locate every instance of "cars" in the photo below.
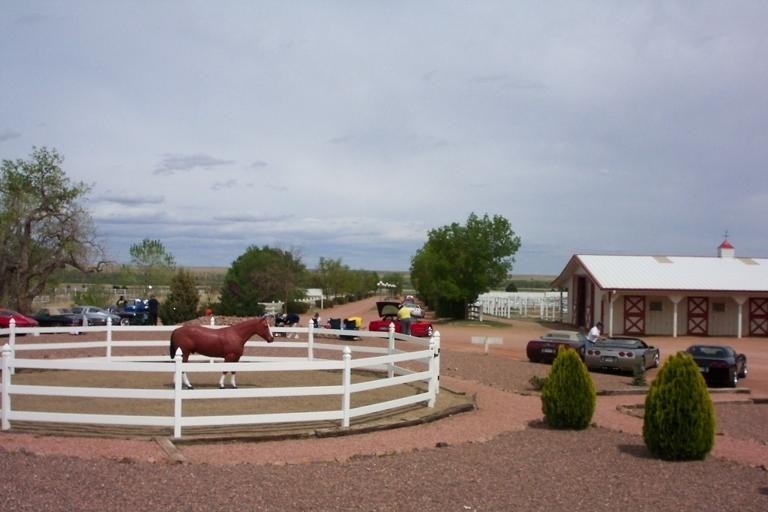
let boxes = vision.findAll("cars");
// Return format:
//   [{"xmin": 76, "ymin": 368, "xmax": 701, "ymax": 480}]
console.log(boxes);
[
  {"xmin": 368, "ymin": 294, "xmax": 434, "ymax": 340},
  {"xmin": 0, "ymin": 298, "xmax": 150, "ymax": 336},
  {"xmin": 525, "ymin": 330, "xmax": 660, "ymax": 376},
  {"xmin": 684, "ymin": 344, "xmax": 748, "ymax": 388}
]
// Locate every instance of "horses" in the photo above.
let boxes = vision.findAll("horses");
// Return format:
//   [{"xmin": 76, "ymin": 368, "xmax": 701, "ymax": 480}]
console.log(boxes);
[{"xmin": 170, "ymin": 316, "xmax": 274, "ymax": 389}]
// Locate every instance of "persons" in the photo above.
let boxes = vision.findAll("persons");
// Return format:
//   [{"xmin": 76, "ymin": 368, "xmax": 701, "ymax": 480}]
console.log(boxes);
[
  {"xmin": 117, "ymin": 296, "xmax": 127, "ymax": 305},
  {"xmin": 397, "ymin": 304, "xmax": 412, "ymax": 335},
  {"xmin": 147, "ymin": 295, "xmax": 158, "ymax": 325},
  {"xmin": 584, "ymin": 322, "xmax": 604, "ymax": 349},
  {"xmin": 205, "ymin": 305, "xmax": 213, "ymax": 317},
  {"xmin": 313, "ymin": 312, "xmax": 320, "ymax": 328},
  {"xmin": 283, "ymin": 313, "xmax": 299, "ymax": 338}
]
